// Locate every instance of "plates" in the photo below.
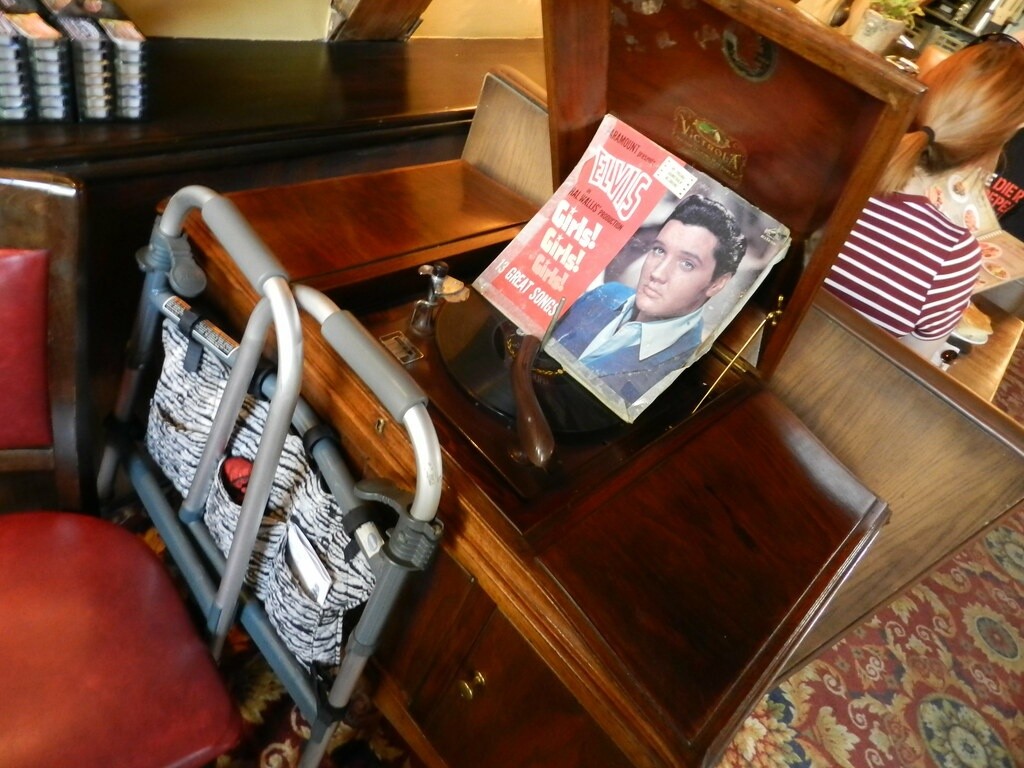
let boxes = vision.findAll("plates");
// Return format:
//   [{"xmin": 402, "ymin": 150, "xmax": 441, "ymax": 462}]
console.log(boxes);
[
  {"xmin": 979, "ymin": 242, "xmax": 1003, "ymax": 260},
  {"xmin": 981, "ymin": 260, "xmax": 1012, "ymax": 281},
  {"xmin": 930, "ymin": 184, "xmax": 944, "ymax": 209},
  {"xmin": 964, "ymin": 204, "xmax": 980, "ymax": 234},
  {"xmin": 949, "ymin": 175, "xmax": 969, "ymax": 203},
  {"xmin": 951, "ymin": 330, "xmax": 988, "ymax": 345}
]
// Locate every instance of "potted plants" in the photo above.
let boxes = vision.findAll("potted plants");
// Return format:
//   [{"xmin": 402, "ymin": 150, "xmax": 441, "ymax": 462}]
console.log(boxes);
[{"xmin": 853, "ymin": 0, "xmax": 926, "ymax": 56}]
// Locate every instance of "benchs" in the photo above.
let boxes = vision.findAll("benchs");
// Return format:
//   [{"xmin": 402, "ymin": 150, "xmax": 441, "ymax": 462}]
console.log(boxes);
[{"xmin": 766, "ymin": 287, "xmax": 1024, "ymax": 686}]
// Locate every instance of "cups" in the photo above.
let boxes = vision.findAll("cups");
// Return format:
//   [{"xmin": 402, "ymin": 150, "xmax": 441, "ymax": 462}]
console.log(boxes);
[{"xmin": 912, "ymin": 43, "xmax": 953, "ymax": 83}]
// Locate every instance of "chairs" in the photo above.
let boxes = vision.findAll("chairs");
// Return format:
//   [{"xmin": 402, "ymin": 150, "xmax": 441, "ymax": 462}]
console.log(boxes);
[{"xmin": 0, "ymin": 168, "xmax": 242, "ymax": 768}]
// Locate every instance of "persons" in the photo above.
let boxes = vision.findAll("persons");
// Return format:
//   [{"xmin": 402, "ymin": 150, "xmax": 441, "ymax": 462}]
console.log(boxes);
[
  {"xmin": 805, "ymin": 32, "xmax": 1024, "ymax": 363},
  {"xmin": 551, "ymin": 192, "xmax": 747, "ymax": 406}
]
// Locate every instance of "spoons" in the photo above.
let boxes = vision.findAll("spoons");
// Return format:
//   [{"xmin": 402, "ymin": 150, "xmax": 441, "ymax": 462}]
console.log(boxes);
[{"xmin": 939, "ymin": 350, "xmax": 959, "ymax": 369}]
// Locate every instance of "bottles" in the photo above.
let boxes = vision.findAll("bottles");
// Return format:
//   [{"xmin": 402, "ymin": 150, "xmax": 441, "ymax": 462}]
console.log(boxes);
[{"xmin": 852, "ymin": 8, "xmax": 907, "ymax": 56}]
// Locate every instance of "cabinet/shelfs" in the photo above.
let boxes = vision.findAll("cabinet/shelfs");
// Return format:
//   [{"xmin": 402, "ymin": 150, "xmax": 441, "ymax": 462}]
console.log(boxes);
[{"xmin": 151, "ymin": 158, "xmax": 889, "ymax": 768}]
